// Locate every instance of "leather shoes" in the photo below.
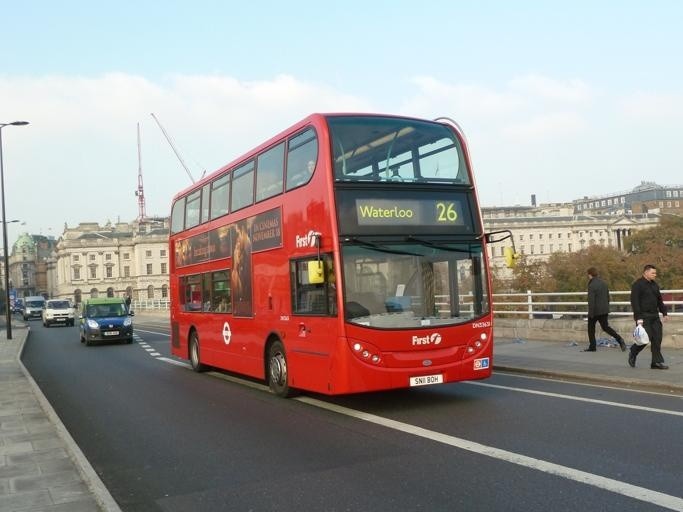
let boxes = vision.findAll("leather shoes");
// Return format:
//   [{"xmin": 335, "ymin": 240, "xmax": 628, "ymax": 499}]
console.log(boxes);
[
  {"xmin": 627, "ymin": 351, "xmax": 637, "ymax": 368},
  {"xmin": 650, "ymin": 361, "xmax": 670, "ymax": 370}
]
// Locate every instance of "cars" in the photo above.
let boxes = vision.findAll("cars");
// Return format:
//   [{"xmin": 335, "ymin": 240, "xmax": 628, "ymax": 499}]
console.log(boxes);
[
  {"xmin": 10, "ymin": 296, "xmax": 77, "ymax": 328},
  {"xmin": 79, "ymin": 297, "xmax": 135, "ymax": 346}
]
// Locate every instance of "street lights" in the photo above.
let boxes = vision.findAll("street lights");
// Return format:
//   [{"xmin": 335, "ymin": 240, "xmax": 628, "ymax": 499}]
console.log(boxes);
[{"xmin": 0, "ymin": 120, "xmax": 30, "ymax": 340}]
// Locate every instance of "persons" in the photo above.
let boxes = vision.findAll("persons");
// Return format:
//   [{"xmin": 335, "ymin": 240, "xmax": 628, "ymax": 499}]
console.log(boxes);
[
  {"xmin": 297, "ymin": 157, "xmax": 316, "ymax": 185},
  {"xmin": 628, "ymin": 265, "xmax": 671, "ymax": 370},
  {"xmin": 583, "ymin": 266, "xmax": 627, "ymax": 353}
]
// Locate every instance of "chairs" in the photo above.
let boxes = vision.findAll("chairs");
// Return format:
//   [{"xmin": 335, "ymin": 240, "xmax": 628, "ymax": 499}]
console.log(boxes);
[{"xmin": 185, "ymin": 267, "xmax": 392, "ymax": 314}]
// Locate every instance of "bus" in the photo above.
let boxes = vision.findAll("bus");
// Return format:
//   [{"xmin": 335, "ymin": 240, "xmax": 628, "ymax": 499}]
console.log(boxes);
[{"xmin": 169, "ymin": 112, "xmax": 516, "ymax": 399}]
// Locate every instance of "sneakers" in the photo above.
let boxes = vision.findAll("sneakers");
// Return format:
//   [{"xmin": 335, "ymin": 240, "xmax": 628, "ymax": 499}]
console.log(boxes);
[{"xmin": 584, "ymin": 338, "xmax": 627, "ymax": 352}]
